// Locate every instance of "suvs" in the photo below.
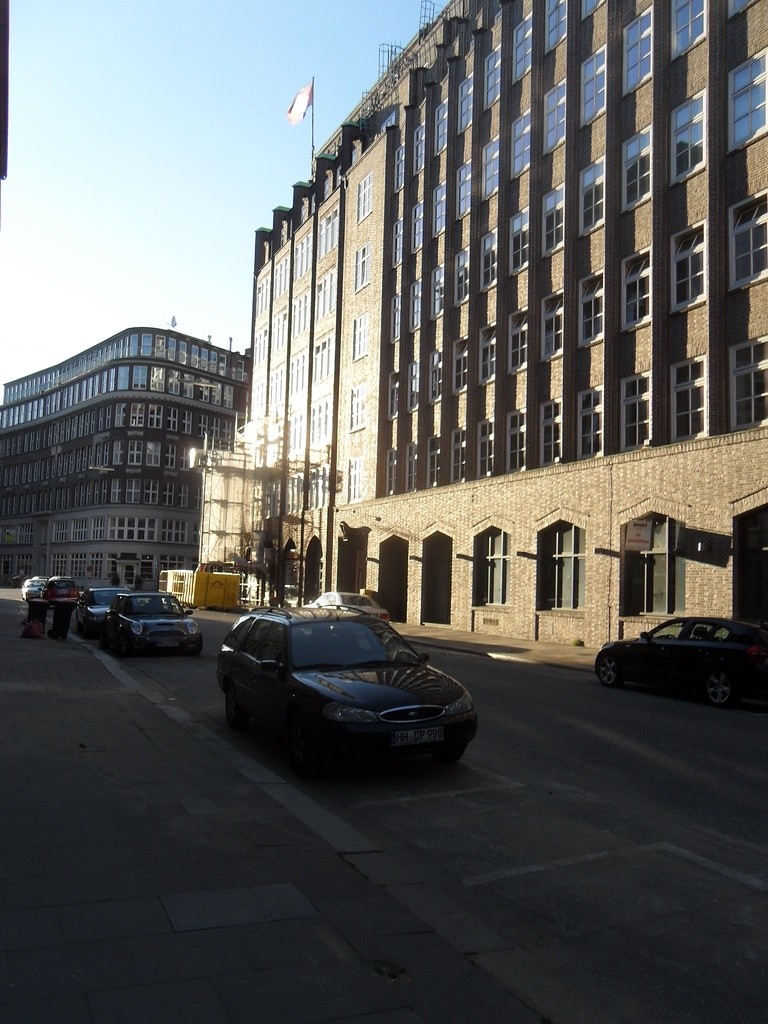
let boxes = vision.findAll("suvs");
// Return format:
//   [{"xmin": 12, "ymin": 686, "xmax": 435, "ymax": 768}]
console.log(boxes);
[{"xmin": 215, "ymin": 605, "xmax": 478, "ymax": 768}]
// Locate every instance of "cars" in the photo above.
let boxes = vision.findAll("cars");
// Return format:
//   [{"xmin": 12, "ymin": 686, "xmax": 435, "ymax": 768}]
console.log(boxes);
[
  {"xmin": 102, "ymin": 593, "xmax": 203, "ymax": 656},
  {"xmin": 297, "ymin": 591, "xmax": 390, "ymax": 625},
  {"xmin": 76, "ymin": 587, "xmax": 131, "ymax": 638},
  {"xmin": 37, "ymin": 575, "xmax": 79, "ymax": 608},
  {"xmin": 29, "ymin": 575, "xmax": 53, "ymax": 584},
  {"xmin": 595, "ymin": 616, "xmax": 768, "ymax": 709},
  {"xmin": 21, "ymin": 579, "xmax": 46, "ymax": 602}
]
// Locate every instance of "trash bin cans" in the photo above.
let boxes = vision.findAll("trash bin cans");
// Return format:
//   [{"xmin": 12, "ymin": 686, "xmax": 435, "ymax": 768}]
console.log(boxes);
[
  {"xmin": 23, "ymin": 597, "xmax": 50, "ymax": 636},
  {"xmin": 47, "ymin": 597, "xmax": 78, "ymax": 640}
]
